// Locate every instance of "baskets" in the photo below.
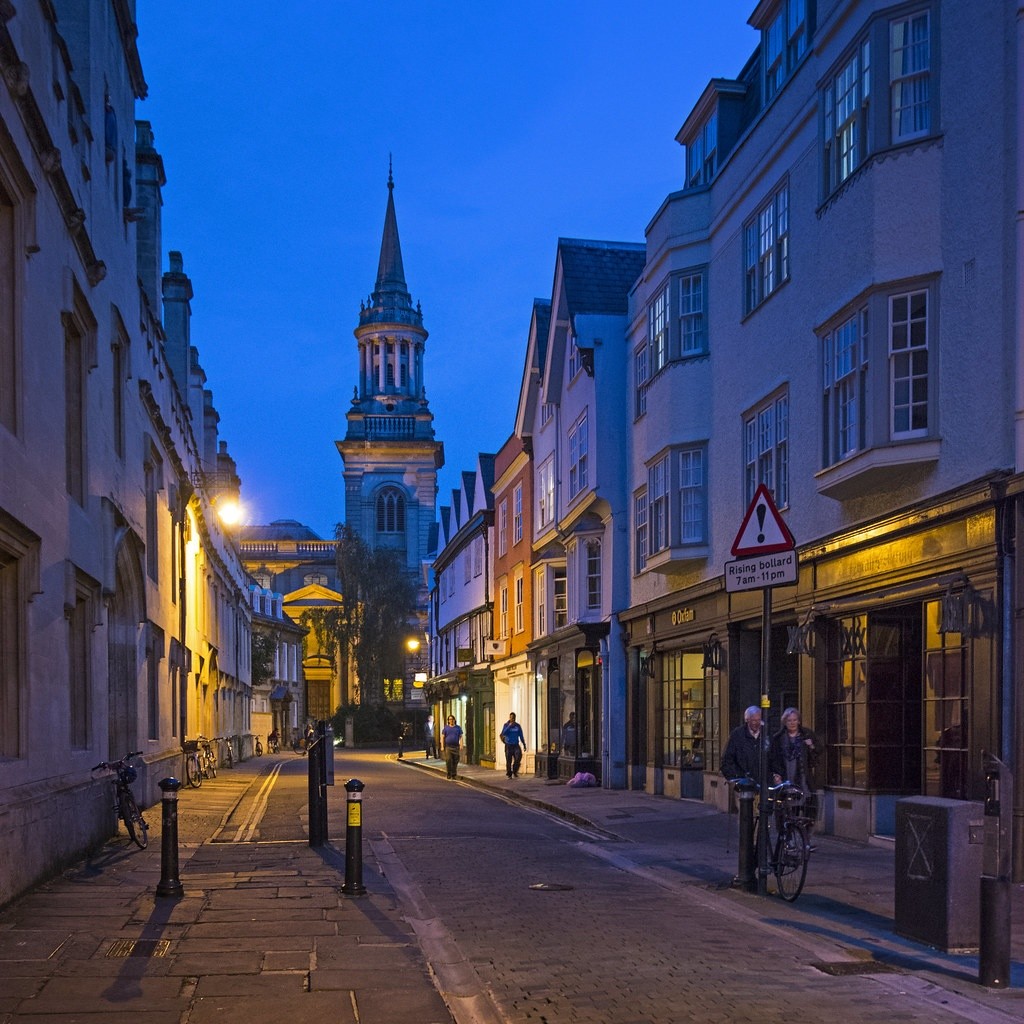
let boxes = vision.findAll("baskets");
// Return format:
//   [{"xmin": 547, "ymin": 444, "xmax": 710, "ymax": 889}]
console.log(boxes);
[
  {"xmin": 781, "ymin": 792, "xmax": 824, "ymax": 820},
  {"xmin": 181, "ymin": 741, "xmax": 198, "ymax": 754}
]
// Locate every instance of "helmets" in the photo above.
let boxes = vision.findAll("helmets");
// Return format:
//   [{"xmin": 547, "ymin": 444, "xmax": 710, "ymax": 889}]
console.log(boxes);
[{"xmin": 122, "ymin": 765, "xmax": 138, "ymax": 784}]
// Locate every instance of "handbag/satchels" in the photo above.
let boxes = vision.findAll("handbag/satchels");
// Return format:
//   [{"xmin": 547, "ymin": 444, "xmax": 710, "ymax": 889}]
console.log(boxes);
[{"xmin": 500, "ymin": 733, "xmax": 506, "ymax": 744}]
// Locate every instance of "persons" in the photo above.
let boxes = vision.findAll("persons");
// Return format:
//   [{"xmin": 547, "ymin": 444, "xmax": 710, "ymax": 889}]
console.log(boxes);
[
  {"xmin": 440, "ymin": 715, "xmax": 464, "ymax": 780},
  {"xmin": 933, "ymin": 720, "xmax": 970, "ymax": 798},
  {"xmin": 269, "ymin": 727, "xmax": 280, "ymax": 754},
  {"xmin": 501, "ymin": 712, "xmax": 527, "ymax": 780},
  {"xmin": 303, "ymin": 724, "xmax": 314, "ymax": 755},
  {"xmin": 720, "ymin": 706, "xmax": 777, "ymax": 850},
  {"xmin": 770, "ymin": 707, "xmax": 824, "ymax": 852},
  {"xmin": 563, "ymin": 711, "xmax": 577, "ymax": 756},
  {"xmin": 424, "ymin": 714, "xmax": 439, "ymax": 760}
]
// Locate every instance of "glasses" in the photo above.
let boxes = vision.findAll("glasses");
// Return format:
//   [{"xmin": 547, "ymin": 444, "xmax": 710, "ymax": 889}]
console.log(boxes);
[{"xmin": 751, "ymin": 720, "xmax": 762, "ymax": 725}]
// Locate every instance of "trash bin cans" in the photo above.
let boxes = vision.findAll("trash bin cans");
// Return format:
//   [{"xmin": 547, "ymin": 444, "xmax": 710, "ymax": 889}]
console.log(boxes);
[{"xmin": 893, "ymin": 795, "xmax": 985, "ymax": 956}]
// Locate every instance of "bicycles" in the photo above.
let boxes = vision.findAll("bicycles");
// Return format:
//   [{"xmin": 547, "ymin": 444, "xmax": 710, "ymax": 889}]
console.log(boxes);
[
  {"xmin": 250, "ymin": 734, "xmax": 264, "ymax": 757},
  {"xmin": 215, "ymin": 734, "xmax": 238, "ymax": 769},
  {"xmin": 179, "ymin": 735, "xmax": 218, "ymax": 789},
  {"xmin": 91, "ymin": 751, "xmax": 149, "ymax": 850},
  {"xmin": 730, "ymin": 775, "xmax": 817, "ymax": 902}
]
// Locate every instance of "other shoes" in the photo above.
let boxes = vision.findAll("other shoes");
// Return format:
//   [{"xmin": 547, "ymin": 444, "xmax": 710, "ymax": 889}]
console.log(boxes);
[
  {"xmin": 509, "ymin": 776, "xmax": 512, "ymax": 779},
  {"xmin": 784, "ymin": 842, "xmax": 797, "ymax": 852},
  {"xmin": 447, "ymin": 776, "xmax": 455, "ymax": 780},
  {"xmin": 514, "ymin": 774, "xmax": 518, "ymax": 777},
  {"xmin": 800, "ymin": 844, "xmax": 817, "ymax": 851}
]
